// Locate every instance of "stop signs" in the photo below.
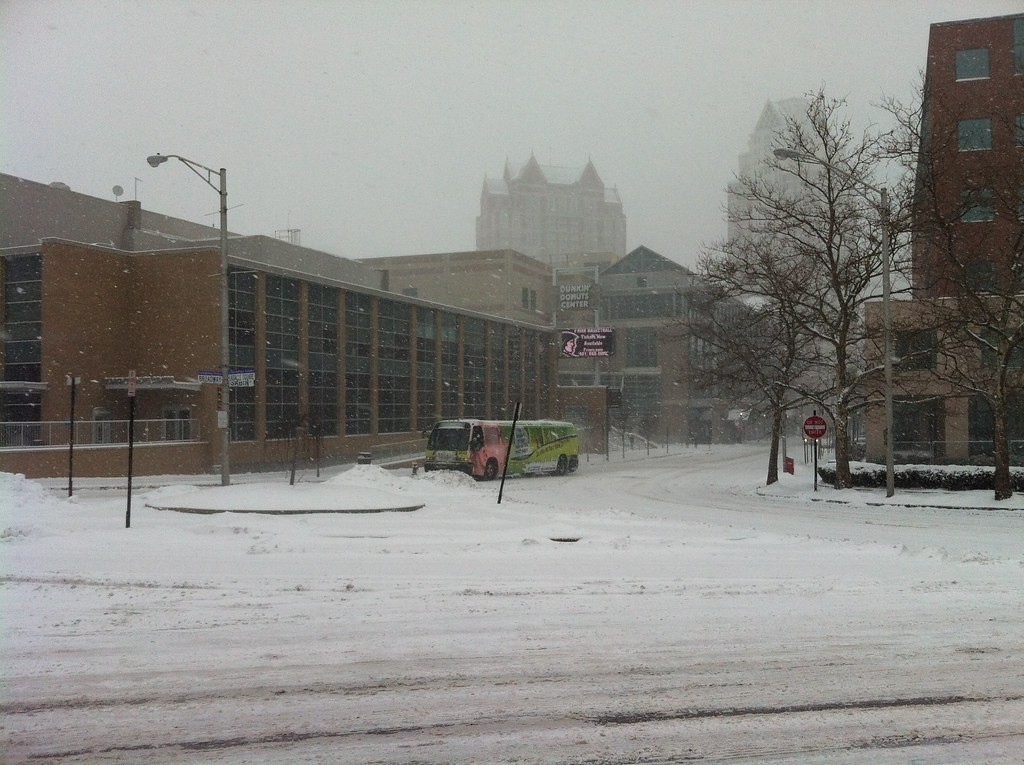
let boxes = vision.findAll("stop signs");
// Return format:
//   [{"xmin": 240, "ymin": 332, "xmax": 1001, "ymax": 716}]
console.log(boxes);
[{"xmin": 803, "ymin": 415, "xmax": 828, "ymax": 439}]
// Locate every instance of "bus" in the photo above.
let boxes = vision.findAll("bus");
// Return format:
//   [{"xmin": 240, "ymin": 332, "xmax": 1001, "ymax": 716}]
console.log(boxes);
[{"xmin": 422, "ymin": 421, "xmax": 580, "ymax": 480}]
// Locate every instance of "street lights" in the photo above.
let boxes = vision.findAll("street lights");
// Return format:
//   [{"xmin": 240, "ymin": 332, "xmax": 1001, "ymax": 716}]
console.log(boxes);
[
  {"xmin": 147, "ymin": 152, "xmax": 235, "ymax": 486},
  {"xmin": 774, "ymin": 148, "xmax": 897, "ymax": 499}
]
